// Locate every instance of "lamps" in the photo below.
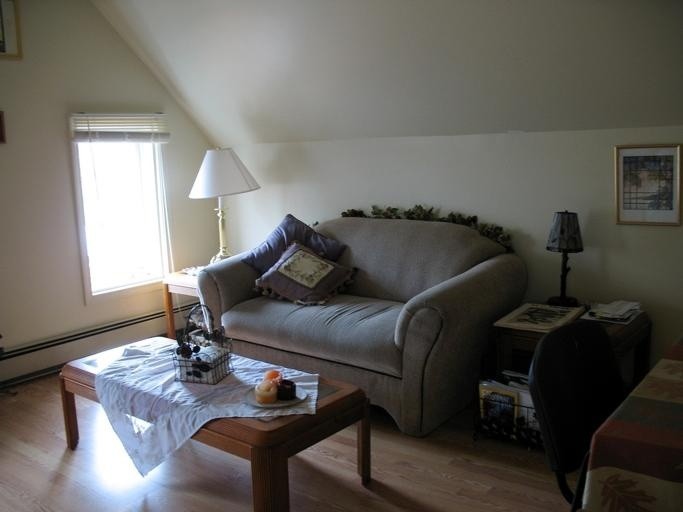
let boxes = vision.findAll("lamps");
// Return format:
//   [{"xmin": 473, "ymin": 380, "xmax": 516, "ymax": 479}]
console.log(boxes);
[
  {"xmin": 545, "ymin": 210, "xmax": 584, "ymax": 307},
  {"xmin": 188, "ymin": 147, "xmax": 261, "ymax": 268}
]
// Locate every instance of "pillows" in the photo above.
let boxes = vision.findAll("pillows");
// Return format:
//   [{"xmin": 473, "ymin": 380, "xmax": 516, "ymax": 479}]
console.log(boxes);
[{"xmin": 242, "ymin": 214, "xmax": 359, "ymax": 307}]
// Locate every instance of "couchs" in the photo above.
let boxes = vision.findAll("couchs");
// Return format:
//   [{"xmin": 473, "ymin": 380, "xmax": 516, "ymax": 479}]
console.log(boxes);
[{"xmin": 195, "ymin": 206, "xmax": 528, "ymax": 439}]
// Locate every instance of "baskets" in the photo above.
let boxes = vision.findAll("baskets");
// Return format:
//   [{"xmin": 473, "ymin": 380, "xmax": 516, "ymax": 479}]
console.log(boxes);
[{"xmin": 173, "ymin": 304, "xmax": 234, "ymax": 385}]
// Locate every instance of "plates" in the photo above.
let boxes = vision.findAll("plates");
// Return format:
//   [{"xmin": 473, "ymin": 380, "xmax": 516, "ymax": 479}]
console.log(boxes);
[{"xmin": 245, "ymin": 384, "xmax": 308, "ymax": 408}]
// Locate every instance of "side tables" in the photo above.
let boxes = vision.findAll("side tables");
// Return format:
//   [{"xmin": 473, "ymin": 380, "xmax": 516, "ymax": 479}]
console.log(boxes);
[
  {"xmin": 162, "ymin": 266, "xmax": 208, "ymax": 340},
  {"xmin": 495, "ymin": 302, "xmax": 653, "ymax": 396}
]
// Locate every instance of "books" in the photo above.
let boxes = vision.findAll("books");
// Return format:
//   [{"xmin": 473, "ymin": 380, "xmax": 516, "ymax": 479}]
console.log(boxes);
[
  {"xmin": 493, "ymin": 301, "xmax": 585, "ymax": 335},
  {"xmin": 477, "ymin": 369, "xmax": 544, "ymax": 446}
]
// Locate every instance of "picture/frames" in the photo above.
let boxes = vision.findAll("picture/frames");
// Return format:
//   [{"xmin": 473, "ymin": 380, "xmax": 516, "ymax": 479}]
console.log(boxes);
[{"xmin": 613, "ymin": 143, "xmax": 682, "ymax": 226}]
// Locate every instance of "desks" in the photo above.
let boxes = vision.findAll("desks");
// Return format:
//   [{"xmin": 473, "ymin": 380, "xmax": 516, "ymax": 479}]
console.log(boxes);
[{"xmin": 582, "ymin": 338, "xmax": 683, "ymax": 512}]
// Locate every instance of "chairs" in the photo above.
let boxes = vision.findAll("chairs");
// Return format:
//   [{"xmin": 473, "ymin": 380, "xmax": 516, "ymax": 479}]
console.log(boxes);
[{"xmin": 529, "ymin": 318, "xmax": 625, "ymax": 504}]
